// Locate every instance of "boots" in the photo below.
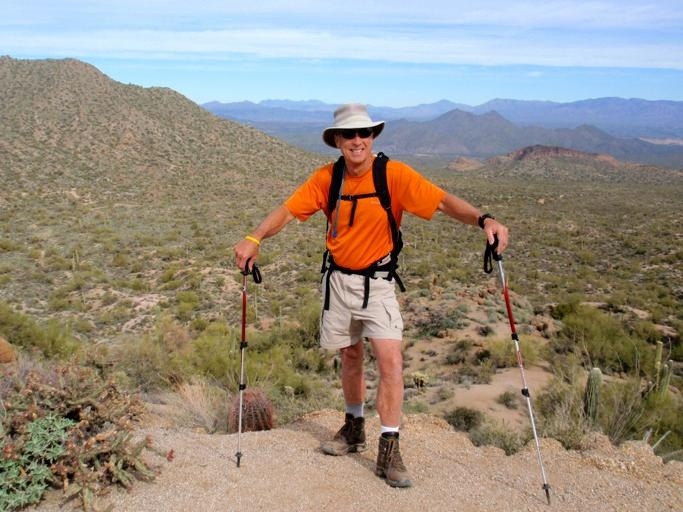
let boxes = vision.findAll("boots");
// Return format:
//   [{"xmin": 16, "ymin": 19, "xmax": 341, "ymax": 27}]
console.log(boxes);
[
  {"xmin": 376, "ymin": 431, "xmax": 411, "ymax": 487},
  {"xmin": 321, "ymin": 413, "xmax": 366, "ymax": 456}
]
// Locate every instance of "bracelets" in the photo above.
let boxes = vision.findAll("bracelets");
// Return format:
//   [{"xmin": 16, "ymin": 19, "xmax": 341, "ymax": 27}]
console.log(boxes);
[{"xmin": 243, "ymin": 233, "xmax": 264, "ymax": 247}]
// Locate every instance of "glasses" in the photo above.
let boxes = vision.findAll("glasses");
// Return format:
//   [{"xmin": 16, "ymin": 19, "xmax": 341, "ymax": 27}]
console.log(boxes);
[{"xmin": 341, "ymin": 127, "xmax": 373, "ymax": 138}]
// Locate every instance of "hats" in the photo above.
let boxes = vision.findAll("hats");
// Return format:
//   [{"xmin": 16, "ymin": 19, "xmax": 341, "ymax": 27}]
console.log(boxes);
[{"xmin": 321, "ymin": 103, "xmax": 385, "ymax": 149}]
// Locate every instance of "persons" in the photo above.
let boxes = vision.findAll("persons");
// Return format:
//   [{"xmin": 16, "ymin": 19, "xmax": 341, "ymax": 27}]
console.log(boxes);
[{"xmin": 234, "ymin": 102, "xmax": 509, "ymax": 488}]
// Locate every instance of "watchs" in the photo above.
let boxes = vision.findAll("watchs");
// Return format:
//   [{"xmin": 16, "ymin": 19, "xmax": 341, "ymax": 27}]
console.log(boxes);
[{"xmin": 478, "ymin": 212, "xmax": 494, "ymax": 229}]
